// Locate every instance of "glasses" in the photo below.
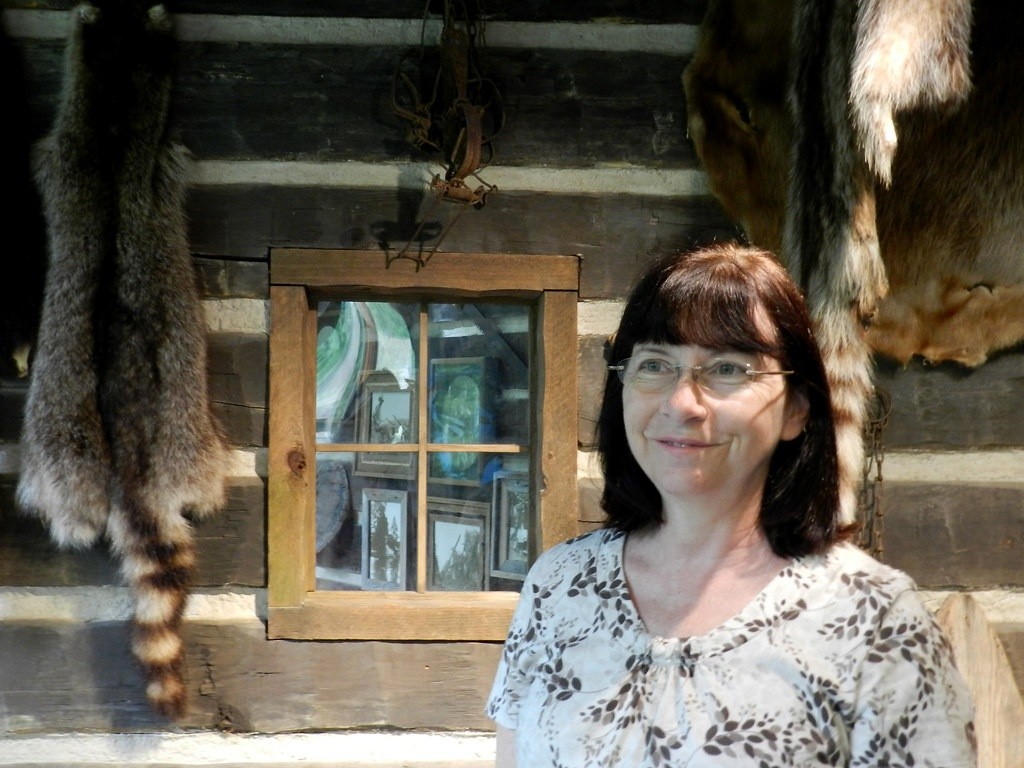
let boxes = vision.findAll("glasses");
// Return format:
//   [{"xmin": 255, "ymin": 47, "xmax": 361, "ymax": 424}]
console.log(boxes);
[{"xmin": 604, "ymin": 358, "xmax": 794, "ymax": 396}]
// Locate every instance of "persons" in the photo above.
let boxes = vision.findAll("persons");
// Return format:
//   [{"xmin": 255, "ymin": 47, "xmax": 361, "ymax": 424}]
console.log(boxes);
[{"xmin": 484, "ymin": 244, "xmax": 977, "ymax": 768}]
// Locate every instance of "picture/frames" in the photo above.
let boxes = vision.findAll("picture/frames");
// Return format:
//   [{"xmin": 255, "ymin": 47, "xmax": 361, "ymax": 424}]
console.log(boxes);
[
  {"xmin": 423, "ymin": 495, "xmax": 490, "ymax": 594},
  {"xmin": 427, "ymin": 354, "xmax": 497, "ymax": 488},
  {"xmin": 352, "ymin": 370, "xmax": 418, "ymax": 481},
  {"xmin": 360, "ymin": 489, "xmax": 407, "ymax": 591},
  {"xmin": 488, "ymin": 470, "xmax": 528, "ymax": 583}
]
